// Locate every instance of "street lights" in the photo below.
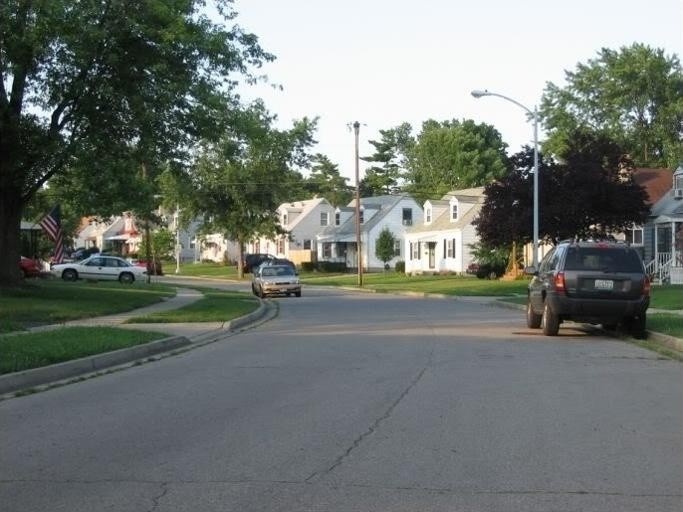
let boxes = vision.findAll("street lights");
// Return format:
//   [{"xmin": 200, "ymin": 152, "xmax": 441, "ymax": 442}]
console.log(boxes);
[
  {"xmin": 472, "ymin": 90, "xmax": 539, "ymax": 275},
  {"xmin": 153, "ymin": 193, "xmax": 181, "ymax": 274}
]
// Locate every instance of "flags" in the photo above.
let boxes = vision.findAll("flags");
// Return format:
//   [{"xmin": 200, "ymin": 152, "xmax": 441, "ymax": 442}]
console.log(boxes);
[{"xmin": 37, "ymin": 202, "xmax": 65, "ymax": 265}]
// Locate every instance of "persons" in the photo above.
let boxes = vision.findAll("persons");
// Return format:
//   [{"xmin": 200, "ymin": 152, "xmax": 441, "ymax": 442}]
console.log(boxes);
[{"xmin": 88, "ymin": 239, "xmax": 99, "ymax": 254}]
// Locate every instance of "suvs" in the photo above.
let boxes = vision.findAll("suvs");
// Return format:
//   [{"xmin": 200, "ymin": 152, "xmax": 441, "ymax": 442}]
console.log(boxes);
[
  {"xmin": 524, "ymin": 238, "xmax": 651, "ymax": 339},
  {"xmin": 237, "ymin": 253, "xmax": 296, "ymax": 273}
]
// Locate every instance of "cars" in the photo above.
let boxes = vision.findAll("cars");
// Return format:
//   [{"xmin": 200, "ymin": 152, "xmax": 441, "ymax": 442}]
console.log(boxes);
[
  {"xmin": 19, "ymin": 245, "xmax": 161, "ymax": 286},
  {"xmin": 466, "ymin": 261, "xmax": 505, "ymax": 280},
  {"xmin": 252, "ymin": 265, "xmax": 301, "ymax": 298}
]
